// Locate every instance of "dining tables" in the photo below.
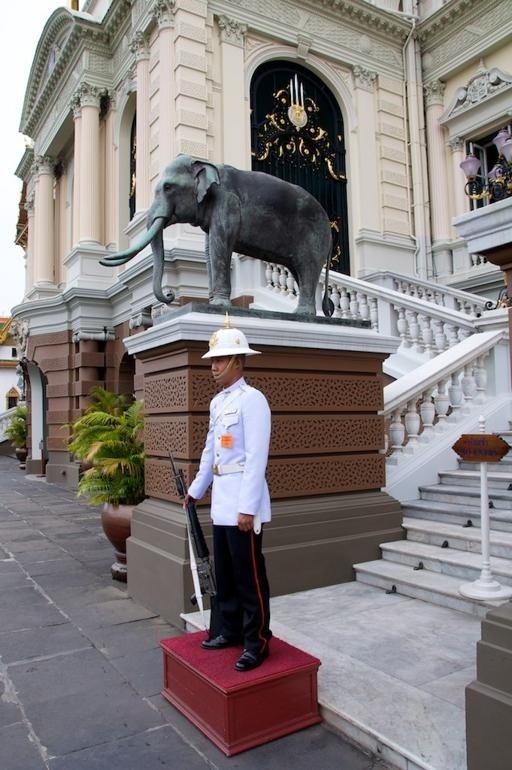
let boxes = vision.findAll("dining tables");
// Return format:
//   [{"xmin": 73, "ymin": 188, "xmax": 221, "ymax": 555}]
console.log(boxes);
[
  {"xmin": 57, "ymin": 381, "xmax": 146, "ymax": 581},
  {"xmin": 3, "ymin": 404, "xmax": 28, "ymax": 469}
]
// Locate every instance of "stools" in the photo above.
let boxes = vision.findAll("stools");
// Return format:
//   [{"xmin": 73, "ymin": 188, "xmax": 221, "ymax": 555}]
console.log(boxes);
[{"xmin": 211, "ymin": 463, "xmax": 245, "ymax": 475}]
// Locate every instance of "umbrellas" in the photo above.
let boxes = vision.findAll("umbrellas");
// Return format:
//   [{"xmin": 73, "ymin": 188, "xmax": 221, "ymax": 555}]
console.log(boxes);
[
  {"xmin": 235, "ymin": 646, "xmax": 270, "ymax": 670},
  {"xmin": 201, "ymin": 635, "xmax": 243, "ymax": 650}
]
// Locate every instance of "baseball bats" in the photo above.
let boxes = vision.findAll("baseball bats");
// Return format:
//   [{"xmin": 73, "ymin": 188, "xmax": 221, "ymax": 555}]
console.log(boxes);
[{"xmin": 166, "ymin": 448, "xmax": 222, "ymax": 641}]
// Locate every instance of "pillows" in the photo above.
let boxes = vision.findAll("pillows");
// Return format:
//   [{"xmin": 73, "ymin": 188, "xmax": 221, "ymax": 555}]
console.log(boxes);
[{"xmin": 201, "ymin": 327, "xmax": 262, "ymax": 359}]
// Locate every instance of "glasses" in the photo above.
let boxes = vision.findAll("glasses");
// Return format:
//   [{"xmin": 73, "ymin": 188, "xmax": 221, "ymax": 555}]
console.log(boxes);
[{"xmin": 459, "ymin": 129, "xmax": 512, "ymax": 201}]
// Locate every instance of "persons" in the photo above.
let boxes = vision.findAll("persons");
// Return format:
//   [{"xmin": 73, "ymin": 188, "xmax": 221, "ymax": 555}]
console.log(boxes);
[{"xmin": 180, "ymin": 326, "xmax": 274, "ymax": 673}]
